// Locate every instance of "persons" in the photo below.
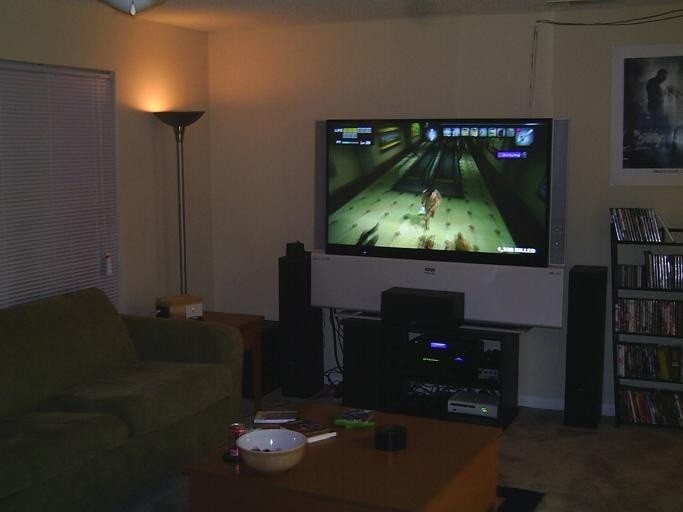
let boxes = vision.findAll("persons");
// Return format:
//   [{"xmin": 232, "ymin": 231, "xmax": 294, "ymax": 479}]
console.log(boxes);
[
  {"xmin": 421, "ymin": 184, "xmax": 441, "ymax": 230},
  {"xmin": 646, "ymin": 68, "xmax": 670, "ymax": 117}
]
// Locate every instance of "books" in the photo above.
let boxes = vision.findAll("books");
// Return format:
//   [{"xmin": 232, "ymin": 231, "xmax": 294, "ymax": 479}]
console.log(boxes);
[
  {"xmin": 608, "ymin": 205, "xmax": 682, "ymax": 429},
  {"xmin": 330, "ymin": 407, "xmax": 377, "ymax": 430},
  {"xmin": 253, "ymin": 410, "xmax": 298, "ymax": 424},
  {"xmin": 280, "ymin": 417, "xmax": 338, "ymax": 443}
]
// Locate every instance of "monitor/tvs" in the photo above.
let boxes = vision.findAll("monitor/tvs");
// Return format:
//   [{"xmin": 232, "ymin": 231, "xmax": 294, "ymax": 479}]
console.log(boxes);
[{"xmin": 309, "ymin": 116, "xmax": 571, "ymax": 330}]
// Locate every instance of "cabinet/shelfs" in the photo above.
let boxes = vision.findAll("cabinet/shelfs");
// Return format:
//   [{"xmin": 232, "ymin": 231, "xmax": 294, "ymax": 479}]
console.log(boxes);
[{"xmin": 610, "ymin": 222, "xmax": 682, "ymax": 430}]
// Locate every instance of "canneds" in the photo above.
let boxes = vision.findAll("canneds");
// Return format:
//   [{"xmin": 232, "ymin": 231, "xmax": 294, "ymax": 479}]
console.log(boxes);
[{"xmin": 230, "ymin": 423, "xmax": 247, "ymax": 456}]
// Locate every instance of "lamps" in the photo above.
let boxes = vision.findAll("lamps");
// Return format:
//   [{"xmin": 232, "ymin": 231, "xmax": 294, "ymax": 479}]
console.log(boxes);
[{"xmin": 154, "ymin": 111, "xmax": 206, "ymax": 293}]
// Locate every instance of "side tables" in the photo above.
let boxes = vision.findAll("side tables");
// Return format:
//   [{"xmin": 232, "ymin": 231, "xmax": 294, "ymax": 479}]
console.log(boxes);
[{"xmin": 201, "ymin": 311, "xmax": 266, "ymax": 410}]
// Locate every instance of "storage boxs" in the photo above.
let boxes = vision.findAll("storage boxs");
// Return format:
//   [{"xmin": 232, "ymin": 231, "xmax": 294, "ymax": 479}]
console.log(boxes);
[{"xmin": 156, "ymin": 295, "xmax": 203, "ymax": 321}]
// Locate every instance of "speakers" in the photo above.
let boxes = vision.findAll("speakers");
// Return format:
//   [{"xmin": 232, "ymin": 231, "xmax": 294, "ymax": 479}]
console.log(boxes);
[
  {"xmin": 561, "ymin": 262, "xmax": 609, "ymax": 427},
  {"xmin": 277, "ymin": 250, "xmax": 325, "ymax": 399},
  {"xmin": 380, "ymin": 287, "xmax": 465, "ymax": 330}
]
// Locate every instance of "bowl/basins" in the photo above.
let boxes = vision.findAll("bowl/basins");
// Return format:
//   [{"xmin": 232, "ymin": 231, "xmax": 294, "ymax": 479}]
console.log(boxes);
[{"xmin": 235, "ymin": 428, "xmax": 307, "ymax": 473}]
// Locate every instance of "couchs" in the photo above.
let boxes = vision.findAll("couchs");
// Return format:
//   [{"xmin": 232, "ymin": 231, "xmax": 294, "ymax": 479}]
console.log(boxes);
[{"xmin": 0, "ymin": 284, "xmax": 244, "ymax": 511}]
